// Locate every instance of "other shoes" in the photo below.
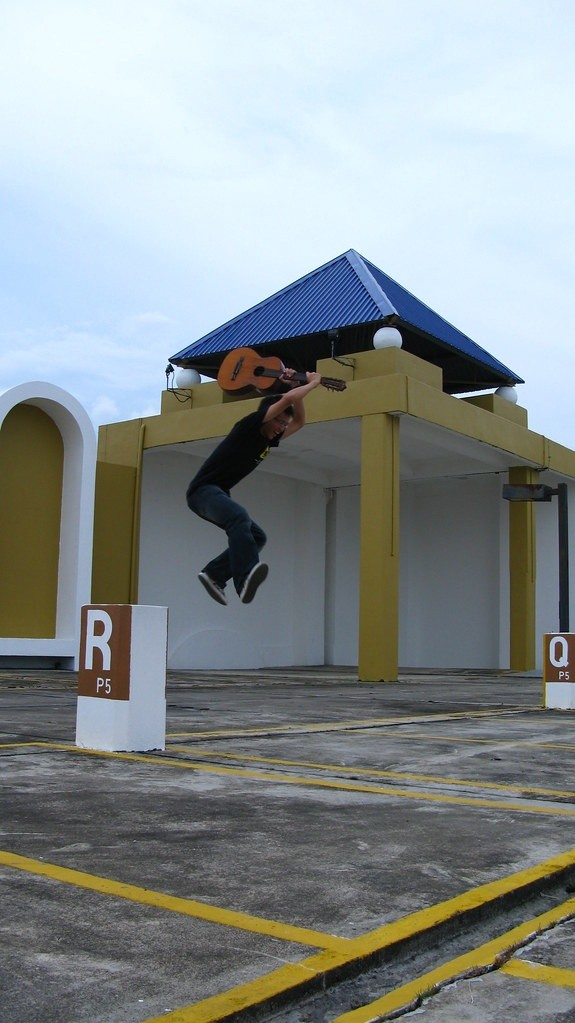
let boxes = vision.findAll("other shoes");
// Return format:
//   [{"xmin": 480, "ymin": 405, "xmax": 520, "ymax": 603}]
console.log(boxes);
[
  {"xmin": 235, "ymin": 562, "xmax": 269, "ymax": 603},
  {"xmin": 198, "ymin": 572, "xmax": 228, "ymax": 606}
]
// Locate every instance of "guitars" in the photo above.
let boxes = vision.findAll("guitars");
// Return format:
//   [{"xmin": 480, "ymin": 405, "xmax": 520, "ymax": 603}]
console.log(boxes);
[{"xmin": 219, "ymin": 348, "xmax": 347, "ymax": 401}]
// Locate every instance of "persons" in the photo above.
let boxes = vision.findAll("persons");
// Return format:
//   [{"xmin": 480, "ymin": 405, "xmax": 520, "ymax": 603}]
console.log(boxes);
[{"xmin": 186, "ymin": 368, "xmax": 320, "ymax": 606}]
